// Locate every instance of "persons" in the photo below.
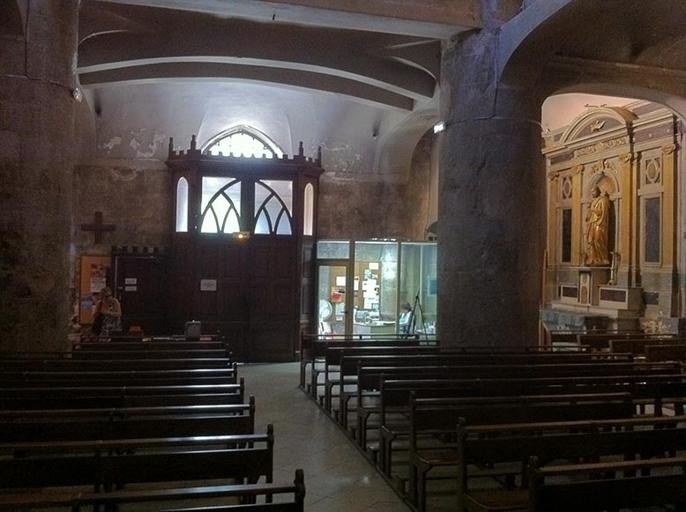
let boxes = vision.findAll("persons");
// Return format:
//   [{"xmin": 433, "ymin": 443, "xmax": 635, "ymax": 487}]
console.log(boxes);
[
  {"xmin": 93, "ymin": 287, "xmax": 122, "ymax": 336},
  {"xmin": 399, "ymin": 303, "xmax": 417, "ymax": 337}
]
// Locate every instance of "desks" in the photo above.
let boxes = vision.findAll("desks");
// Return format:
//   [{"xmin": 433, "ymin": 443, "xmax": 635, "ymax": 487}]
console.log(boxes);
[{"xmin": 353, "ymin": 320, "xmax": 397, "ymax": 339}]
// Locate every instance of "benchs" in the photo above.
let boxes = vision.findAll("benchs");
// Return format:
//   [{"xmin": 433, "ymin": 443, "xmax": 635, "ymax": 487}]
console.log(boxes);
[
  {"xmin": 0, "ymin": 329, "xmax": 305, "ymax": 512},
  {"xmin": 298, "ymin": 329, "xmax": 686, "ymax": 512}
]
